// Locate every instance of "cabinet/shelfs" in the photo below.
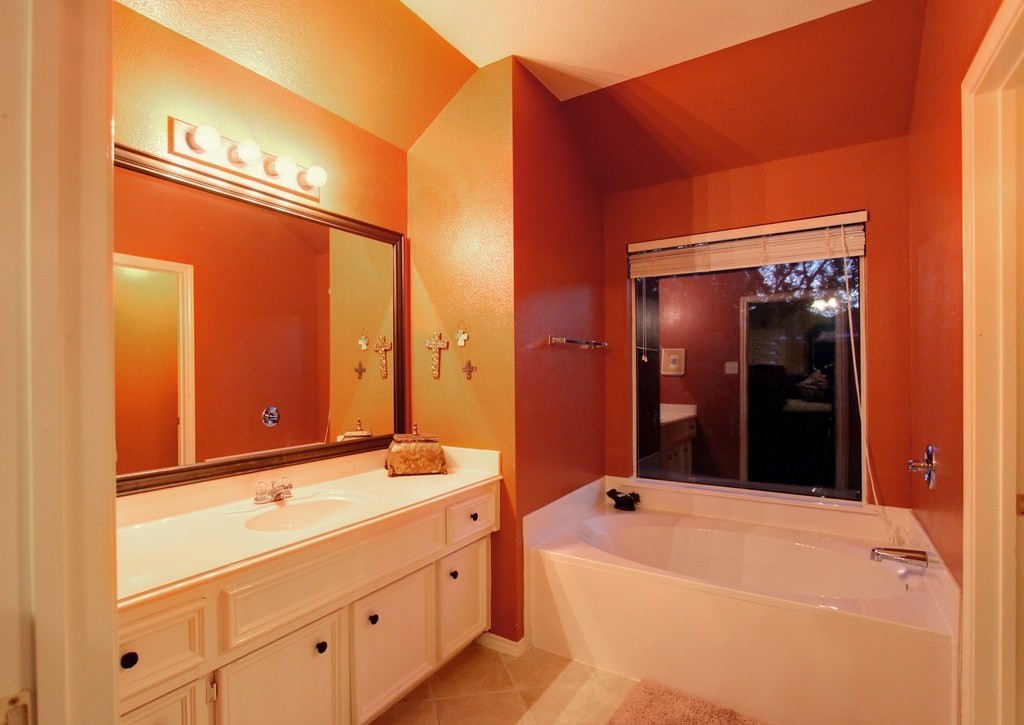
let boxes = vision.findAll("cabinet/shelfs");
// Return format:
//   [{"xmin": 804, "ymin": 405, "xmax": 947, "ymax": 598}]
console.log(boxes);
[
  {"xmin": 660, "ymin": 415, "xmax": 697, "ymax": 477},
  {"xmin": 118, "ymin": 479, "xmax": 502, "ymax": 724}
]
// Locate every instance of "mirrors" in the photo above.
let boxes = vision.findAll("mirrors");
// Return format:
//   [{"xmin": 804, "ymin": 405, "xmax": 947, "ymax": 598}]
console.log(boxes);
[{"xmin": 113, "ymin": 141, "xmax": 405, "ymax": 498}]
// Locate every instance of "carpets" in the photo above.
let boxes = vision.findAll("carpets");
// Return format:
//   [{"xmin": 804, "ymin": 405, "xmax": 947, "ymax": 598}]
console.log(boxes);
[{"xmin": 605, "ymin": 678, "xmax": 767, "ymax": 725}]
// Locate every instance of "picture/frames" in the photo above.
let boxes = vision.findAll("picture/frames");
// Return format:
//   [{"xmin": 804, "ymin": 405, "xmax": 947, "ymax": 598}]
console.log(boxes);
[{"xmin": 661, "ymin": 348, "xmax": 685, "ymax": 376}]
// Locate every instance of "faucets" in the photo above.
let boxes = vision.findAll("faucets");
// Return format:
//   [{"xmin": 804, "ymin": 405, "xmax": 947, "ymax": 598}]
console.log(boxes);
[
  {"xmin": 869, "ymin": 546, "xmax": 928, "ymax": 568},
  {"xmin": 252, "ymin": 475, "xmax": 294, "ymax": 506}
]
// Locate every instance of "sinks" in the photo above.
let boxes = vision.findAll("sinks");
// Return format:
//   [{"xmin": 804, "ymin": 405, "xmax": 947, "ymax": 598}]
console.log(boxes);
[{"xmin": 243, "ymin": 497, "xmax": 363, "ymax": 536}]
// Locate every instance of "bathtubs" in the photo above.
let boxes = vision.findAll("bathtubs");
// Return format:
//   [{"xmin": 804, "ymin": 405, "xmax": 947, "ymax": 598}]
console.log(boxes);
[{"xmin": 529, "ymin": 503, "xmax": 952, "ymax": 725}]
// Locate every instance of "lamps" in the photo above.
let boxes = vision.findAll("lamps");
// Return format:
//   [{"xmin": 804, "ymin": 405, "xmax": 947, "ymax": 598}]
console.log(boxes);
[{"xmin": 168, "ymin": 116, "xmax": 327, "ymax": 202}]
